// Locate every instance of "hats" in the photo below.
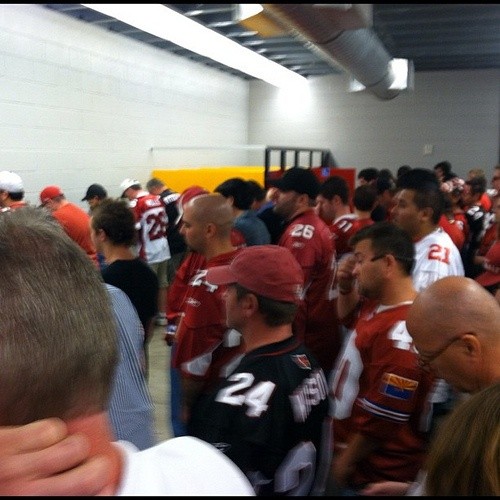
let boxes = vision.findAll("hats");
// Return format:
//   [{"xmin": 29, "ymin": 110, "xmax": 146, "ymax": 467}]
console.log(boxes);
[
  {"xmin": 81, "ymin": 183, "xmax": 109, "ymax": 202},
  {"xmin": 38, "ymin": 185, "xmax": 64, "ymax": 209},
  {"xmin": 0, "ymin": 170, "xmax": 25, "ymax": 194},
  {"xmin": 206, "ymin": 244, "xmax": 306, "ymax": 304},
  {"xmin": 118, "ymin": 178, "xmax": 140, "ymax": 200},
  {"xmin": 172, "ymin": 185, "xmax": 210, "ymax": 229},
  {"xmin": 265, "ymin": 167, "xmax": 321, "ymax": 201}
]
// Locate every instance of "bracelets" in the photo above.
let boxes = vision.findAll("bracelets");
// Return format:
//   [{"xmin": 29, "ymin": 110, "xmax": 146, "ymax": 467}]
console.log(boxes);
[
  {"xmin": 339, "ymin": 283, "xmax": 353, "ymax": 291},
  {"xmin": 338, "ymin": 289, "xmax": 353, "ymax": 295}
]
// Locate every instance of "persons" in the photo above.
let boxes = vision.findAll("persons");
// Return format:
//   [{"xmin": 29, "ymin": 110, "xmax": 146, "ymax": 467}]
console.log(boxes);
[
  {"xmin": 189, "ymin": 246, "xmax": 333, "ymax": 497},
  {"xmin": 357, "ymin": 276, "xmax": 500, "ymax": 498},
  {"xmin": 330, "ymin": 221, "xmax": 439, "ymax": 498},
  {"xmin": 0, "ymin": 207, "xmax": 257, "ymax": 497},
  {"xmin": 1, "ymin": 161, "xmax": 500, "ymax": 468}
]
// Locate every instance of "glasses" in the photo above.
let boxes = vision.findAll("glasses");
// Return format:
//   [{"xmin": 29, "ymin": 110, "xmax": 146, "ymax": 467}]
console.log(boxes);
[
  {"xmin": 371, "ymin": 255, "xmax": 416, "ymax": 275},
  {"xmin": 407, "ymin": 330, "xmax": 476, "ymax": 377}
]
